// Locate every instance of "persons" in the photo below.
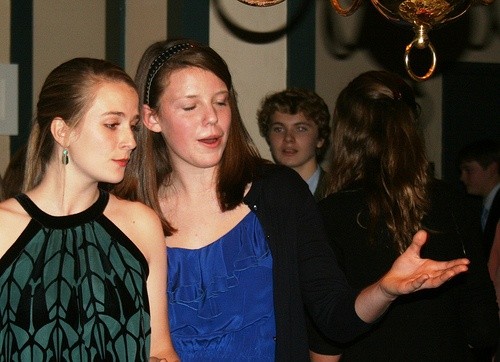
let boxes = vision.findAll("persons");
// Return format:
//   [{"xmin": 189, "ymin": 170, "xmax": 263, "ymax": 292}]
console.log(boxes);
[
  {"xmin": 257, "ymin": 86, "xmax": 331, "ymax": 202},
  {"xmin": 0, "ymin": 58, "xmax": 180, "ymax": 362},
  {"xmin": 309, "ymin": 69, "xmax": 500, "ymax": 362},
  {"xmin": 459, "ymin": 139, "xmax": 500, "ymax": 253},
  {"xmin": 105, "ymin": 39, "xmax": 498, "ymax": 362}
]
